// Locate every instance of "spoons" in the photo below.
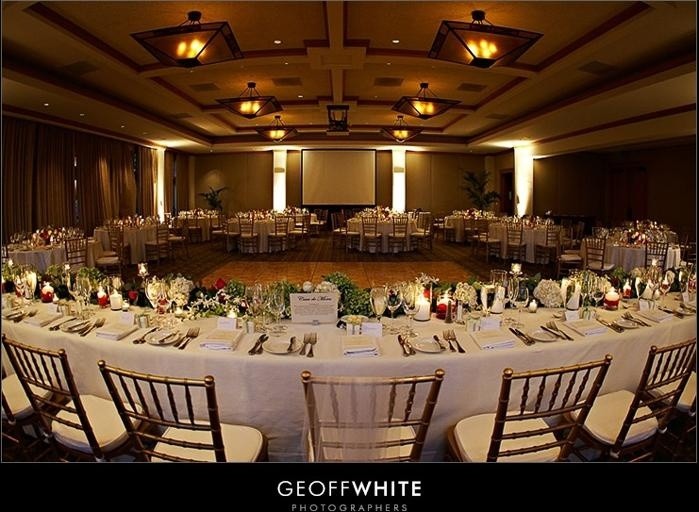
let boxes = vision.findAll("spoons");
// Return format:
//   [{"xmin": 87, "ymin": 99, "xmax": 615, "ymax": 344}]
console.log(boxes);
[{"xmin": 397, "ymin": 335, "xmax": 417, "ymax": 357}]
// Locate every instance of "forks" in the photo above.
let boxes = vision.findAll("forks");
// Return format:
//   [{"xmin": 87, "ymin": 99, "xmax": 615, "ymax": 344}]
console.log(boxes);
[
  {"xmin": 307, "ymin": 331, "xmax": 318, "ymax": 356},
  {"xmin": 442, "ymin": 329, "xmax": 456, "ymax": 353},
  {"xmin": 449, "ymin": 331, "xmax": 466, "ymax": 353},
  {"xmin": 177, "ymin": 326, "xmax": 201, "ymax": 350},
  {"xmin": 300, "ymin": 332, "xmax": 311, "ymax": 354}
]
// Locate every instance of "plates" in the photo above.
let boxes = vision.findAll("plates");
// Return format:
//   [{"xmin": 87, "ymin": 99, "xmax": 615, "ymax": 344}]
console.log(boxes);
[
  {"xmin": 411, "ymin": 338, "xmax": 447, "ymax": 353},
  {"xmin": 146, "ymin": 329, "xmax": 180, "ymax": 346},
  {"xmin": 261, "ymin": 337, "xmax": 303, "ymax": 353},
  {"xmin": 341, "ymin": 315, "xmax": 369, "ymax": 324}
]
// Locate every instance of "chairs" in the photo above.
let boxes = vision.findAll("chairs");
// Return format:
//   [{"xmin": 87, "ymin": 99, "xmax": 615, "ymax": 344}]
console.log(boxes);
[{"xmin": 0, "ymin": 203, "xmax": 699, "ymax": 463}]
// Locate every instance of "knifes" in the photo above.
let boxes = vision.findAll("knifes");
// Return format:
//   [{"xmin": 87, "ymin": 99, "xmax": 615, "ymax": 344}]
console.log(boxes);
[
  {"xmin": 432, "ymin": 336, "xmax": 446, "ymax": 350},
  {"xmin": 133, "ymin": 326, "xmax": 159, "ymax": 343},
  {"xmin": 248, "ymin": 332, "xmax": 268, "ymax": 355},
  {"xmin": 289, "ymin": 335, "xmax": 297, "ymax": 352}
]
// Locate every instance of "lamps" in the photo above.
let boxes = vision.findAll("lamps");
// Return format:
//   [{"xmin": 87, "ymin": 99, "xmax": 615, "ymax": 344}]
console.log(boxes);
[
  {"xmin": 391, "ymin": 81, "xmax": 462, "ymax": 120},
  {"xmin": 215, "ymin": 81, "xmax": 283, "ymax": 120},
  {"xmin": 426, "ymin": 9, "xmax": 544, "ymax": 69},
  {"xmin": 377, "ymin": 115, "xmax": 427, "ymax": 144},
  {"xmin": 255, "ymin": 115, "xmax": 302, "ymax": 145},
  {"xmin": 128, "ymin": 10, "xmax": 246, "ymax": 70}
]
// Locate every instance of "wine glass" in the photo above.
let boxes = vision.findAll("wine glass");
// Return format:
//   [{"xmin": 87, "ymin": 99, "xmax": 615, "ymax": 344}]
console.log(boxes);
[
  {"xmin": 9, "ymin": 225, "xmax": 84, "ymax": 251},
  {"xmin": 370, "ymin": 271, "xmax": 698, "ymax": 346},
  {"xmin": 101, "ymin": 213, "xmax": 155, "ymax": 231},
  {"xmin": 167, "ymin": 199, "xmax": 557, "ymax": 229},
  {"xmin": 595, "ymin": 218, "xmax": 677, "ymax": 250},
  {"xmin": 0, "ymin": 264, "xmax": 286, "ymax": 336}
]
